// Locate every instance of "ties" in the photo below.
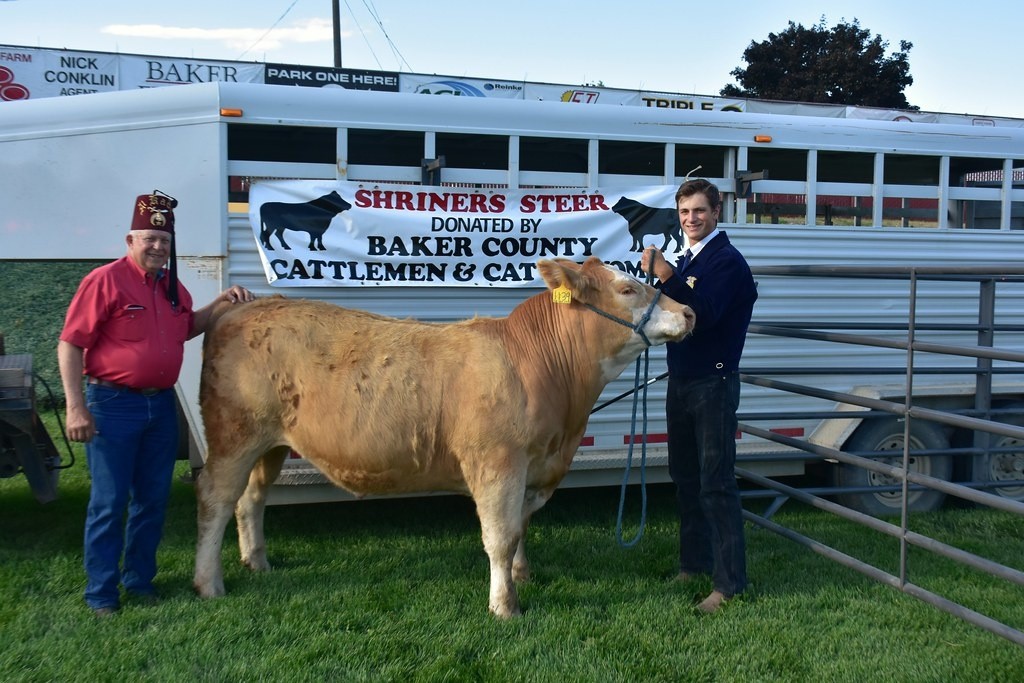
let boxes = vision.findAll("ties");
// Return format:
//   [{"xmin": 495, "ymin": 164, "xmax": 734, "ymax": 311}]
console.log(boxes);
[{"xmin": 677, "ymin": 249, "xmax": 694, "ymax": 279}]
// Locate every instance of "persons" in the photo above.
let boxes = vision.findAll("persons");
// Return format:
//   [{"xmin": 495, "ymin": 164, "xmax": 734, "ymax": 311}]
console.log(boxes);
[
  {"xmin": 641, "ymin": 178, "xmax": 759, "ymax": 612},
  {"xmin": 55, "ymin": 209, "xmax": 256, "ymax": 619}
]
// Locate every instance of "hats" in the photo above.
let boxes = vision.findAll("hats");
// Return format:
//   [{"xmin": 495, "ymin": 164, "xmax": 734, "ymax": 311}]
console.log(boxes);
[{"xmin": 130, "ymin": 195, "xmax": 175, "ymax": 235}]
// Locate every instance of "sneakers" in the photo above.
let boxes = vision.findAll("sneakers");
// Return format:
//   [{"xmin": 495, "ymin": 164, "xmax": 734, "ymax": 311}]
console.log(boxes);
[
  {"xmin": 693, "ymin": 588, "xmax": 735, "ymax": 619},
  {"xmin": 665, "ymin": 567, "xmax": 699, "ymax": 591}
]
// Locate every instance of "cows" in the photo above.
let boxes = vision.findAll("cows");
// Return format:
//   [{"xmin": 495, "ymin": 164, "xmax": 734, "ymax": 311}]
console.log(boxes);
[
  {"xmin": 611, "ymin": 197, "xmax": 685, "ymax": 253},
  {"xmin": 190, "ymin": 254, "xmax": 697, "ymax": 620},
  {"xmin": 259, "ymin": 190, "xmax": 352, "ymax": 252}
]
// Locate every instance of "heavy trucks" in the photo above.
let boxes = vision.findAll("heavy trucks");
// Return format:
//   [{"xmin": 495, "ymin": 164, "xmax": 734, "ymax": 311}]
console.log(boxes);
[{"xmin": 0, "ymin": 81, "xmax": 1022, "ymax": 516}]
